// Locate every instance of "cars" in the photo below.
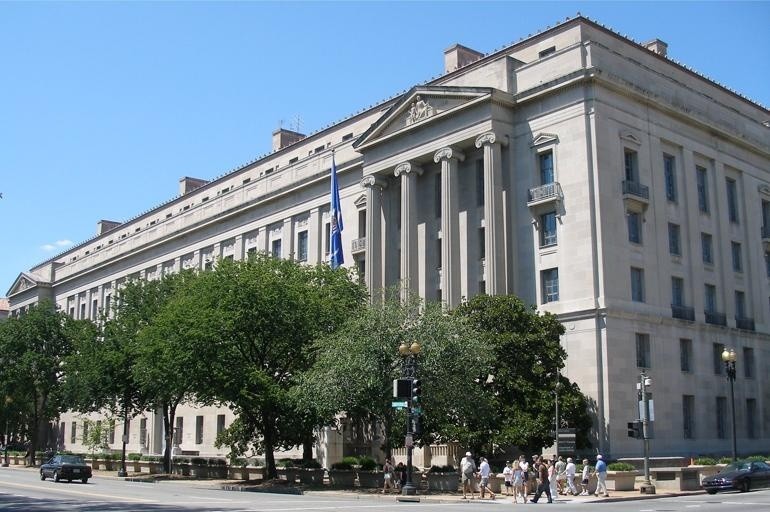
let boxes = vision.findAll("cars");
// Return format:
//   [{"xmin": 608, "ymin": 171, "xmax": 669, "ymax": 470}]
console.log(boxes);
[
  {"xmin": 39, "ymin": 453, "xmax": 93, "ymax": 484},
  {"xmin": 702, "ymin": 460, "xmax": 770, "ymax": 495}
]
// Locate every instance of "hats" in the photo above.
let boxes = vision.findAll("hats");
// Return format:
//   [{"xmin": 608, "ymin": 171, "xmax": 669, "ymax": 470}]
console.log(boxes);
[
  {"xmin": 506, "ymin": 453, "xmax": 605, "ymax": 466},
  {"xmin": 465, "ymin": 450, "xmax": 472, "ymax": 457}
]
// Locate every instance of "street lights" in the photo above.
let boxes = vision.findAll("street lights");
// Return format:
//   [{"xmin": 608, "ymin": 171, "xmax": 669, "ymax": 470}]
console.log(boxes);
[
  {"xmin": 720, "ymin": 348, "xmax": 742, "ymax": 463},
  {"xmin": 1, "ymin": 394, "xmax": 13, "ymax": 466},
  {"xmin": 397, "ymin": 339, "xmax": 422, "ymax": 495},
  {"xmin": 550, "ymin": 380, "xmax": 565, "ymax": 461},
  {"xmin": 635, "ymin": 371, "xmax": 656, "ymax": 493}
]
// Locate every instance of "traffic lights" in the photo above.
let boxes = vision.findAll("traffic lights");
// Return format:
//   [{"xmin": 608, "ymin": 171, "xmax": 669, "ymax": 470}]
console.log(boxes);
[
  {"xmin": 627, "ymin": 420, "xmax": 642, "ymax": 440},
  {"xmin": 409, "ymin": 378, "xmax": 422, "ymax": 407}
]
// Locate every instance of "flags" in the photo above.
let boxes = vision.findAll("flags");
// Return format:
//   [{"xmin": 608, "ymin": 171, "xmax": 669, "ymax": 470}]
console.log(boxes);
[{"xmin": 329, "ymin": 160, "xmax": 344, "ymax": 268}]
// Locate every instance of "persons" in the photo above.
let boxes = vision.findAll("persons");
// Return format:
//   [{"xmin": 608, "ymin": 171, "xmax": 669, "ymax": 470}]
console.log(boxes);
[
  {"xmin": 408, "ymin": 95, "xmax": 428, "ymax": 122},
  {"xmin": 380, "ymin": 459, "xmax": 406, "ymax": 493},
  {"xmin": 460, "ymin": 451, "xmax": 609, "ymax": 503}
]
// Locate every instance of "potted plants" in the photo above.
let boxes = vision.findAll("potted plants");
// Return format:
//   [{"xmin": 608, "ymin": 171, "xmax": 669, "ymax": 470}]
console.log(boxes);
[
  {"xmin": 358, "ymin": 457, "xmax": 385, "ymax": 491},
  {"xmin": 602, "ymin": 462, "xmax": 640, "ymax": 493},
  {"xmin": 468, "ymin": 465, "xmax": 503, "ymax": 494},
  {"xmin": 328, "ymin": 461, "xmax": 358, "ymax": 487},
  {"xmin": 425, "ymin": 463, "xmax": 461, "ymax": 492},
  {"xmin": 495, "ymin": 465, "xmax": 535, "ymax": 498},
  {"xmin": 83, "ymin": 450, "xmax": 327, "ymax": 486},
  {"xmin": 687, "ymin": 458, "xmax": 717, "ymax": 485},
  {"xmin": 392, "ymin": 465, "xmax": 423, "ymax": 487},
  {"xmin": 715, "ymin": 455, "xmax": 741, "ymax": 472},
  {"xmin": 567, "ymin": 464, "xmax": 598, "ymax": 492}
]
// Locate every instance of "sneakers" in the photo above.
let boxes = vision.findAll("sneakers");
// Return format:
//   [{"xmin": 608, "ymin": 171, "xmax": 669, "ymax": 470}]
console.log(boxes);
[
  {"xmin": 461, "ymin": 494, "xmax": 495, "ymax": 500},
  {"xmin": 507, "ymin": 494, "xmax": 552, "ymax": 504},
  {"xmin": 559, "ymin": 491, "xmax": 609, "ymax": 497}
]
